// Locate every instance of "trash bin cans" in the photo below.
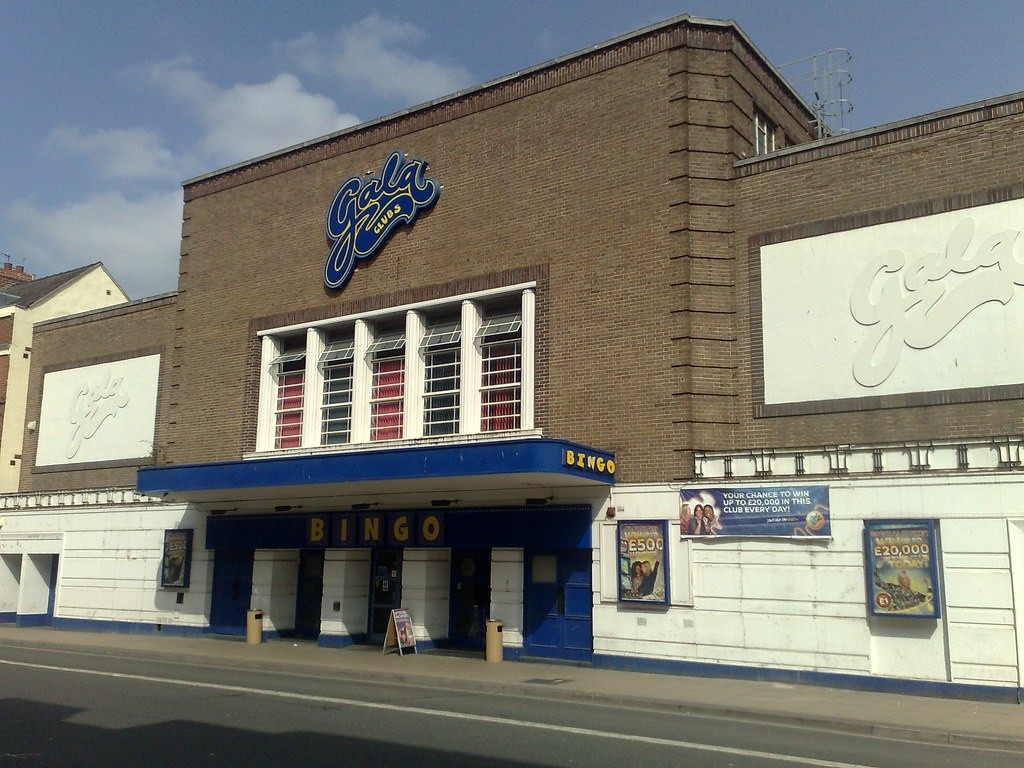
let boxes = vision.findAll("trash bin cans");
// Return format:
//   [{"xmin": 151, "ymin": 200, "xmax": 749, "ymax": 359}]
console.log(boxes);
[
  {"xmin": 247, "ymin": 609, "xmax": 263, "ymax": 645},
  {"xmin": 486, "ymin": 618, "xmax": 504, "ymax": 662}
]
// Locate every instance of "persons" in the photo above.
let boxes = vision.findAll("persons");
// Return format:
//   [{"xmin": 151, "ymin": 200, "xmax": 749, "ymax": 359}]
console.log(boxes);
[
  {"xmin": 680, "ymin": 504, "xmax": 722, "ymax": 535},
  {"xmin": 400, "ymin": 631, "xmax": 410, "ymax": 646},
  {"xmin": 631, "ymin": 554, "xmax": 662, "ymax": 597},
  {"xmin": 875, "ymin": 561, "xmax": 932, "ymax": 610}
]
